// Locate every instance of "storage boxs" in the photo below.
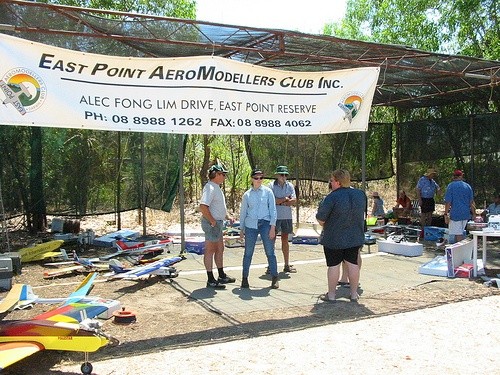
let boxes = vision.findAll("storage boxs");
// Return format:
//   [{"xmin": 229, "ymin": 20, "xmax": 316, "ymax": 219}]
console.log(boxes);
[
  {"xmin": 93, "ymin": 229, "xmax": 139, "ymax": 246},
  {"xmin": 445, "ymin": 238, "xmax": 475, "ymax": 277},
  {"xmin": 418, "ymin": 255, "xmax": 448, "ymax": 277}
]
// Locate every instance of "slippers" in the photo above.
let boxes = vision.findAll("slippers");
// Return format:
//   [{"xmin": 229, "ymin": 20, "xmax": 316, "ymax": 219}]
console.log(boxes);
[
  {"xmin": 337, "ymin": 280, "xmax": 350, "ymax": 285},
  {"xmin": 321, "ymin": 293, "xmax": 337, "ymax": 302},
  {"xmin": 349, "ymin": 293, "xmax": 360, "ymax": 301}
]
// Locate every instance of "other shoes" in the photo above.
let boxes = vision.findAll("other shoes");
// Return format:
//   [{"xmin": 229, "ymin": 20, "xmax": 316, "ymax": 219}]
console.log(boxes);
[
  {"xmin": 272, "ymin": 276, "xmax": 278, "ymax": 288},
  {"xmin": 284, "ymin": 265, "xmax": 297, "ymax": 273},
  {"xmin": 266, "ymin": 266, "xmax": 272, "ymax": 274},
  {"xmin": 207, "ymin": 281, "xmax": 226, "ymax": 289},
  {"xmin": 218, "ymin": 274, "xmax": 236, "ymax": 283},
  {"xmin": 242, "ymin": 278, "xmax": 249, "ymax": 288}
]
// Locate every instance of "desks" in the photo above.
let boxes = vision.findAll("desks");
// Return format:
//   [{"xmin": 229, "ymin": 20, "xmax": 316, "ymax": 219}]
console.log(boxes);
[{"xmin": 469, "ymin": 231, "xmax": 500, "ymax": 277}]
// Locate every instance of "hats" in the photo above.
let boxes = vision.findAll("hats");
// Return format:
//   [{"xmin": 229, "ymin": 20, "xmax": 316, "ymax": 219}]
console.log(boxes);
[
  {"xmin": 209, "ymin": 165, "xmax": 229, "ymax": 173},
  {"xmin": 372, "ymin": 191, "xmax": 380, "ymax": 197},
  {"xmin": 251, "ymin": 170, "xmax": 264, "ymax": 176},
  {"xmin": 454, "ymin": 170, "xmax": 464, "ymax": 176},
  {"xmin": 423, "ymin": 168, "xmax": 436, "ymax": 176},
  {"xmin": 274, "ymin": 166, "xmax": 289, "ymax": 175}
]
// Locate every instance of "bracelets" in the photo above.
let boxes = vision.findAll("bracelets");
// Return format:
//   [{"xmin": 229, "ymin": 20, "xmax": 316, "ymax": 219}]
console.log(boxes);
[{"xmin": 444, "ymin": 214, "xmax": 447, "ymax": 215}]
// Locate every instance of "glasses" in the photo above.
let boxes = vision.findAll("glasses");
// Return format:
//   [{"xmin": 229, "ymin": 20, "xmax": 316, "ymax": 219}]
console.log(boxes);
[
  {"xmin": 220, "ymin": 173, "xmax": 226, "ymax": 176},
  {"xmin": 280, "ymin": 174, "xmax": 288, "ymax": 177},
  {"xmin": 252, "ymin": 176, "xmax": 263, "ymax": 180}
]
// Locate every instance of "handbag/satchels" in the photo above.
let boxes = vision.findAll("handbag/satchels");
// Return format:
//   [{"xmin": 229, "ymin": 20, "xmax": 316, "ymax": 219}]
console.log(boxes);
[{"xmin": 362, "ymin": 190, "xmax": 368, "ymax": 232}]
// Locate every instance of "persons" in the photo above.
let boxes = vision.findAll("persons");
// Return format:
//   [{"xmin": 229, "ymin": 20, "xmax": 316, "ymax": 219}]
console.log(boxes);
[
  {"xmin": 316, "ymin": 169, "xmax": 366, "ymax": 303},
  {"xmin": 395, "ymin": 190, "xmax": 412, "ymax": 213},
  {"xmin": 486, "ymin": 194, "xmax": 500, "ymax": 215},
  {"xmin": 372, "ymin": 191, "xmax": 384, "ymax": 215},
  {"xmin": 239, "ymin": 169, "xmax": 279, "ymax": 288},
  {"xmin": 415, "ymin": 169, "xmax": 441, "ymax": 226},
  {"xmin": 266, "ymin": 166, "xmax": 297, "ymax": 273},
  {"xmin": 200, "ymin": 164, "xmax": 236, "ymax": 289},
  {"xmin": 445, "ymin": 171, "xmax": 476, "ymax": 242}
]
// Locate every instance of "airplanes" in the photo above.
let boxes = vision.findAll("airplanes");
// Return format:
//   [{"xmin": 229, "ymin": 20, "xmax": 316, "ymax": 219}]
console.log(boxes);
[
  {"xmin": 0, "ymin": 239, "xmax": 183, "ymax": 320},
  {"xmin": 0, "ymin": 301, "xmax": 121, "ymax": 375}
]
